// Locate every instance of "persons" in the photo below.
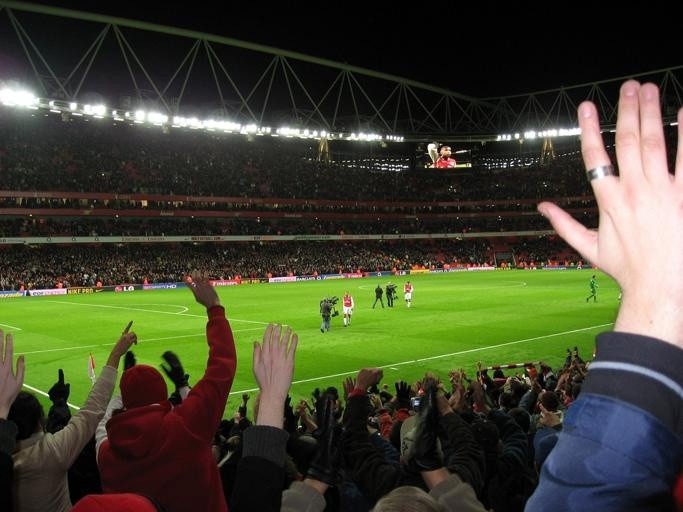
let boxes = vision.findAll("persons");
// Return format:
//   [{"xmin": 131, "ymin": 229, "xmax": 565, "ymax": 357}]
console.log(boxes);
[
  {"xmin": 342, "ymin": 291, "xmax": 354, "ymax": 327},
  {"xmin": 373, "ymin": 284, "xmax": 384, "ymax": 309},
  {"xmin": 428, "ymin": 141, "xmax": 457, "ymax": 168},
  {"xmin": 526, "ymin": 79, "xmax": 682, "ymax": 510},
  {"xmin": 386, "ymin": 284, "xmax": 397, "ymax": 307},
  {"xmin": 2, "ymin": 271, "xmax": 596, "ymax": 510},
  {"xmin": 320, "ymin": 299, "xmax": 331, "ymax": 333},
  {"xmin": 1, "ymin": 104, "xmax": 679, "ymax": 292},
  {"xmin": 586, "ymin": 275, "xmax": 599, "ymax": 302},
  {"xmin": 404, "ymin": 281, "xmax": 414, "ymax": 307},
  {"xmin": 617, "ymin": 288, "xmax": 623, "ymax": 300}
]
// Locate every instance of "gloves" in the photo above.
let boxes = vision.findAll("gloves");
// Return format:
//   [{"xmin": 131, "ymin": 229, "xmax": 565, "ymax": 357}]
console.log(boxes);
[
  {"xmin": 407, "ymin": 383, "xmax": 444, "ymax": 473},
  {"xmin": 395, "ymin": 380, "xmax": 411, "ymax": 409},
  {"xmin": 161, "ymin": 351, "xmax": 189, "ymax": 389},
  {"xmin": 284, "ymin": 394, "xmax": 298, "ymax": 424},
  {"xmin": 47, "ymin": 369, "xmax": 70, "ymax": 407},
  {"xmin": 124, "ymin": 351, "xmax": 137, "ymax": 370},
  {"xmin": 316, "ymin": 388, "xmax": 347, "ymax": 488}
]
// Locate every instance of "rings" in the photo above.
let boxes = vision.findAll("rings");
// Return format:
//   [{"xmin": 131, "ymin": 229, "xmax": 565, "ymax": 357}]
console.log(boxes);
[{"xmin": 587, "ymin": 165, "xmax": 615, "ymax": 180}]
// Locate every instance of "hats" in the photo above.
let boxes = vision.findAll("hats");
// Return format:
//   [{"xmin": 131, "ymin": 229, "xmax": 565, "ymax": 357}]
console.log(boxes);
[
  {"xmin": 120, "ymin": 365, "xmax": 168, "ymax": 406},
  {"xmin": 534, "ymin": 427, "xmax": 558, "ymax": 465}
]
